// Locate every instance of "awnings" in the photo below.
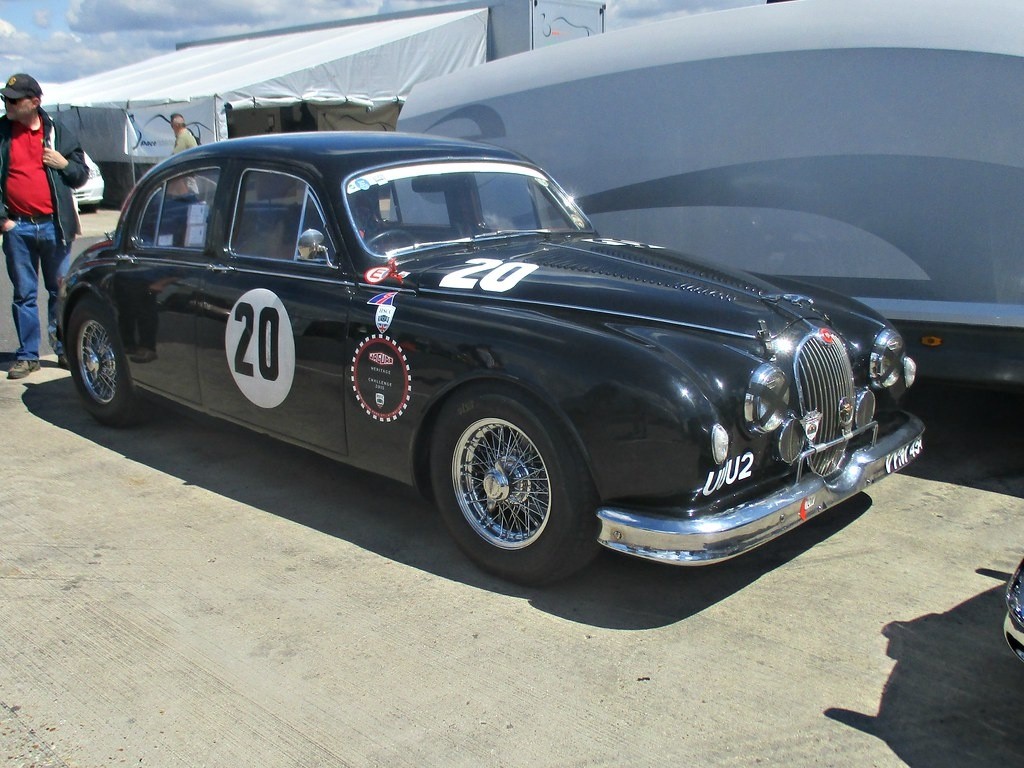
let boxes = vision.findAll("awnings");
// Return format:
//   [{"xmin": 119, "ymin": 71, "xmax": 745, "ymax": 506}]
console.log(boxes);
[{"xmin": 2, "ymin": 7, "xmax": 490, "ymax": 209}]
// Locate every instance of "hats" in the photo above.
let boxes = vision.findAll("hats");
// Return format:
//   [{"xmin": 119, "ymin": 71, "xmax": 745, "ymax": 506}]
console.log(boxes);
[{"xmin": 0, "ymin": 73, "xmax": 42, "ymax": 99}]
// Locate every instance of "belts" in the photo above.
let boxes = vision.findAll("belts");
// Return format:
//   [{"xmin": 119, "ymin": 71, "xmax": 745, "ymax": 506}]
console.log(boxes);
[{"xmin": 9, "ymin": 214, "xmax": 57, "ymax": 224}]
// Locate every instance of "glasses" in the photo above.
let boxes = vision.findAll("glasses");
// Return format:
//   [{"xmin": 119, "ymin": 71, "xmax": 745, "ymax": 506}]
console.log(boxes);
[{"xmin": 0, "ymin": 95, "xmax": 31, "ymax": 105}]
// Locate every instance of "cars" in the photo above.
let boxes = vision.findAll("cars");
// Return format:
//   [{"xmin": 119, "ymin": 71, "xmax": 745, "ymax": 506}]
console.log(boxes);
[
  {"xmin": 67, "ymin": 149, "xmax": 105, "ymax": 214},
  {"xmin": 59, "ymin": 130, "xmax": 927, "ymax": 588}
]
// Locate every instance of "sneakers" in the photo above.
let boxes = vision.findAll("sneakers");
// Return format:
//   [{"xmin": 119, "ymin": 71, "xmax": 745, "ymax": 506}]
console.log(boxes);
[
  {"xmin": 124, "ymin": 346, "xmax": 136, "ymax": 354},
  {"xmin": 131, "ymin": 347, "xmax": 157, "ymax": 363},
  {"xmin": 48, "ymin": 332, "xmax": 71, "ymax": 369},
  {"xmin": 8, "ymin": 361, "xmax": 40, "ymax": 379}
]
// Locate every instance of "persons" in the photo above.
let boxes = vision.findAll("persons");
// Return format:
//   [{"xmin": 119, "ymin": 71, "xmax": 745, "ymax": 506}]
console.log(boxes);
[
  {"xmin": 0, "ymin": 74, "xmax": 90, "ymax": 380},
  {"xmin": 170, "ymin": 113, "xmax": 208, "ymax": 206}
]
